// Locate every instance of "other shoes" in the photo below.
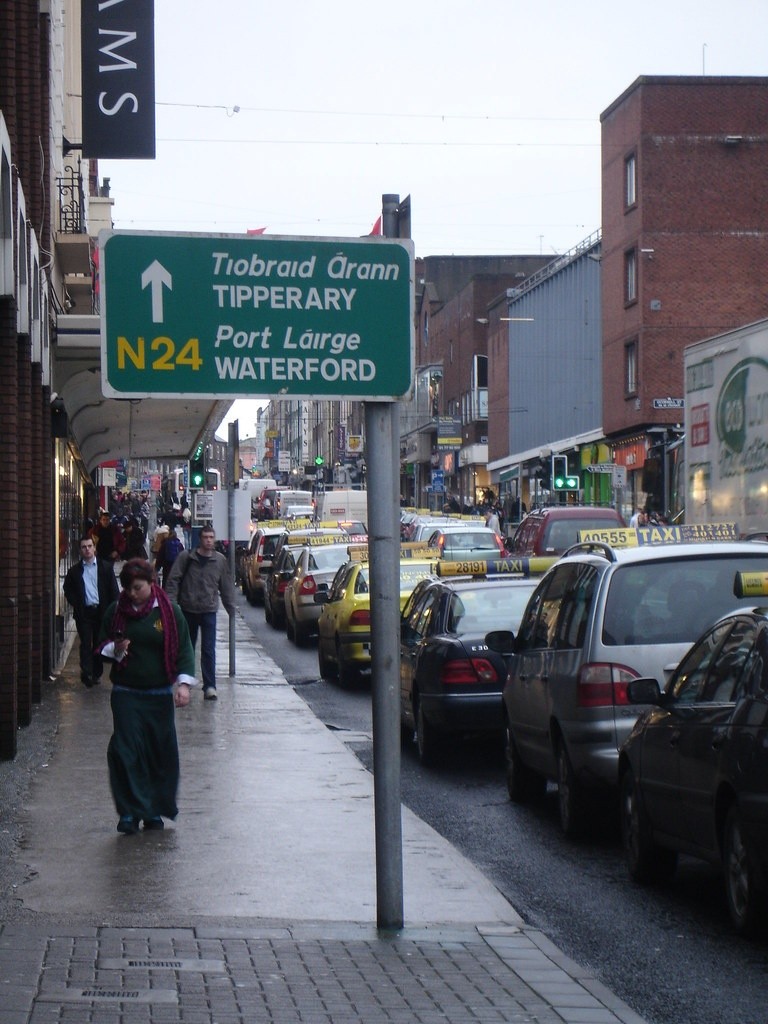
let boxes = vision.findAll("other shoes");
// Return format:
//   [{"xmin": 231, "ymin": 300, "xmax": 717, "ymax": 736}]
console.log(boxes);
[
  {"xmin": 144, "ymin": 815, "xmax": 163, "ymax": 829},
  {"xmin": 117, "ymin": 815, "xmax": 139, "ymax": 834},
  {"xmin": 204, "ymin": 688, "xmax": 217, "ymax": 699},
  {"xmin": 80, "ymin": 671, "xmax": 98, "ymax": 684}
]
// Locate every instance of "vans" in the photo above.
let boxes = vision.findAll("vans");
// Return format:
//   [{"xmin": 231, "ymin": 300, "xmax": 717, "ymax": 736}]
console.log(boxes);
[
  {"xmin": 503, "ymin": 508, "xmax": 630, "ymax": 560},
  {"xmin": 395, "ymin": 556, "xmax": 564, "ymax": 769}
]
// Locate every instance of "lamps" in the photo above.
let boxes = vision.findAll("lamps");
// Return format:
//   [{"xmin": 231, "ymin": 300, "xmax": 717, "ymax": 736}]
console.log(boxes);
[
  {"xmin": 642, "ymin": 247, "xmax": 655, "ymax": 262},
  {"xmin": 587, "ymin": 253, "xmax": 601, "ymax": 263},
  {"xmin": 724, "ymin": 135, "xmax": 743, "ymax": 145}
]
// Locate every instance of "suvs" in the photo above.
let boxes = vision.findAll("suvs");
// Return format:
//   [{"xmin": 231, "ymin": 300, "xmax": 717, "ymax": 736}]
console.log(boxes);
[{"xmin": 484, "ymin": 522, "xmax": 768, "ymax": 844}]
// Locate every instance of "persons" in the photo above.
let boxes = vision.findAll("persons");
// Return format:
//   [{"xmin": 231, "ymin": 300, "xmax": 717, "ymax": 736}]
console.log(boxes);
[
  {"xmin": 108, "ymin": 489, "xmax": 150, "ymax": 520},
  {"xmin": 511, "ymin": 496, "xmax": 528, "ymax": 522},
  {"xmin": 90, "ymin": 559, "xmax": 199, "ymax": 833},
  {"xmin": 464, "ymin": 501, "xmax": 506, "ymax": 537},
  {"xmin": 62, "ymin": 535, "xmax": 120, "ymax": 688},
  {"xmin": 163, "ymin": 526, "xmax": 237, "ymax": 700},
  {"xmin": 150, "ymin": 521, "xmax": 170, "ymax": 573},
  {"xmin": 399, "ymin": 492, "xmax": 409, "ymax": 506},
  {"xmin": 86, "ymin": 511, "xmax": 149, "ymax": 563},
  {"xmin": 630, "ymin": 508, "xmax": 669, "ymax": 528},
  {"xmin": 156, "ymin": 476, "xmax": 193, "ymax": 549},
  {"xmin": 154, "ymin": 528, "xmax": 183, "ymax": 589}
]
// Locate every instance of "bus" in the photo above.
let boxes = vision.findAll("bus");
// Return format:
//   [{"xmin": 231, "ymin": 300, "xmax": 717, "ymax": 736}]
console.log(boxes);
[{"xmin": 168, "ymin": 468, "xmax": 222, "ymax": 512}]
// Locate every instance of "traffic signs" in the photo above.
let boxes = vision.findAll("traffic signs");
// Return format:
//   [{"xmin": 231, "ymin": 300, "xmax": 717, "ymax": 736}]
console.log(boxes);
[{"xmin": 101, "ymin": 228, "xmax": 416, "ymax": 405}]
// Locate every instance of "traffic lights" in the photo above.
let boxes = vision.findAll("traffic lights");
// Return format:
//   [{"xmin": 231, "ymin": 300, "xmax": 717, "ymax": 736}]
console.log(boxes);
[
  {"xmin": 538, "ymin": 456, "xmax": 552, "ymax": 492},
  {"xmin": 189, "ymin": 452, "xmax": 205, "ymax": 492},
  {"xmin": 314, "ymin": 455, "xmax": 323, "ymax": 471},
  {"xmin": 551, "ymin": 454, "xmax": 579, "ymax": 492}
]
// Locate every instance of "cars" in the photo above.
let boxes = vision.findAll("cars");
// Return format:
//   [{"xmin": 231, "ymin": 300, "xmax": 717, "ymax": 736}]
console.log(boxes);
[
  {"xmin": 617, "ymin": 569, "xmax": 768, "ymax": 945},
  {"xmin": 225, "ymin": 477, "xmax": 515, "ymax": 687}
]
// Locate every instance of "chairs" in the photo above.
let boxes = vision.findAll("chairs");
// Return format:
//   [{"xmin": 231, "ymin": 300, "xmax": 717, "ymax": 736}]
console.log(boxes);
[{"xmin": 666, "ymin": 582, "xmax": 704, "ymax": 637}]
